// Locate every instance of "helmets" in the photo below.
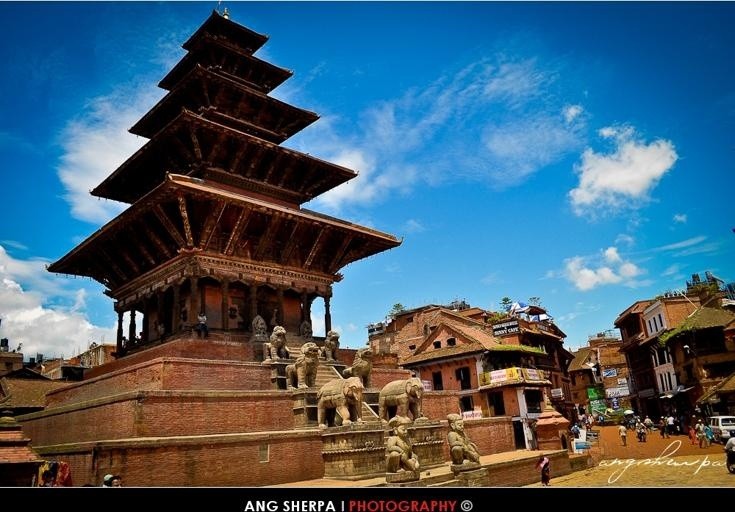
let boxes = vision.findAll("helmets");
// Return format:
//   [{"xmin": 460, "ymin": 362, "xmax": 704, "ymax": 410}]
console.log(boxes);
[{"xmin": 730, "ymin": 430, "xmax": 735, "ymax": 437}]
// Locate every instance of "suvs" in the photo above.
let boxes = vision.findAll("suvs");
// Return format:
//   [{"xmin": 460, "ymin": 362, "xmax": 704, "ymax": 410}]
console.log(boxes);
[{"xmin": 708, "ymin": 415, "xmax": 735, "ymax": 444}]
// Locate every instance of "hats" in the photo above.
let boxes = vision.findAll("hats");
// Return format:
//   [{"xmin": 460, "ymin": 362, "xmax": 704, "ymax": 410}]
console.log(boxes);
[
  {"xmin": 637, "ymin": 419, "xmax": 641, "ymax": 423},
  {"xmin": 103, "ymin": 474, "xmax": 113, "ymax": 481}
]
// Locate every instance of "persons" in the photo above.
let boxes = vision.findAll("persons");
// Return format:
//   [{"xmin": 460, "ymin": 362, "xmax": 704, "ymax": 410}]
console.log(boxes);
[
  {"xmin": 102, "ymin": 474, "xmax": 122, "ymax": 486},
  {"xmin": 447, "ymin": 413, "xmax": 481, "ymax": 463},
  {"xmin": 115, "ymin": 320, "xmax": 170, "ymax": 349},
  {"xmin": 193, "ymin": 311, "xmax": 210, "ymax": 339},
  {"xmin": 533, "ymin": 452, "xmax": 551, "ymax": 486},
  {"xmin": 385, "ymin": 415, "xmax": 420, "ymax": 472},
  {"xmin": 567, "ymin": 400, "xmax": 735, "ymax": 471}
]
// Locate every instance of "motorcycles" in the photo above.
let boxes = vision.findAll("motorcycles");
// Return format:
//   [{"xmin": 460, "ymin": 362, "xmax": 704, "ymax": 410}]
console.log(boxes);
[
  {"xmin": 636, "ymin": 425, "xmax": 647, "ymax": 442},
  {"xmin": 723, "ymin": 446, "xmax": 735, "ymax": 474}
]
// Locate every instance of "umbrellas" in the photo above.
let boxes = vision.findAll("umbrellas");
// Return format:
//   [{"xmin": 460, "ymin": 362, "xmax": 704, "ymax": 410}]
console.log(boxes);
[{"xmin": 506, "ymin": 302, "xmax": 553, "ymax": 323}]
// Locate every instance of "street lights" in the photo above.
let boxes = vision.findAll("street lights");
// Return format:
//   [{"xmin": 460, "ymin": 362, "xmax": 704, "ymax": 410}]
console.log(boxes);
[{"xmin": 682, "ymin": 344, "xmax": 710, "ymax": 424}]
